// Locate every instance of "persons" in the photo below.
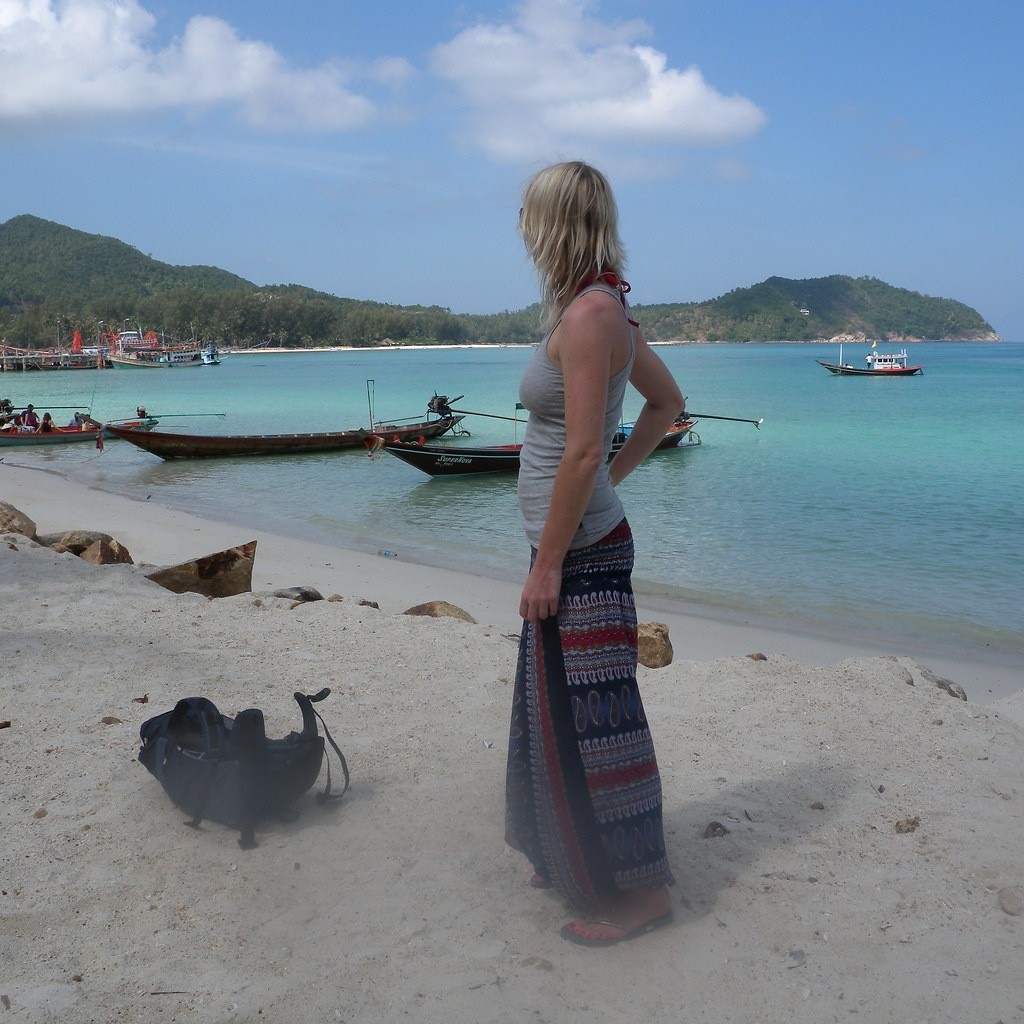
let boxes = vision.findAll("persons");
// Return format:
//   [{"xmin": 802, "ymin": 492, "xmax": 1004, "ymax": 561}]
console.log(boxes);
[
  {"xmin": 69, "ymin": 412, "xmax": 82, "ymax": 426},
  {"xmin": 18, "ymin": 404, "xmax": 40, "ymax": 427},
  {"xmin": 504, "ymin": 162, "xmax": 685, "ymax": 947},
  {"xmin": 82, "ymin": 414, "xmax": 90, "ymax": 430},
  {"xmin": 35, "ymin": 413, "xmax": 63, "ymax": 433},
  {"xmin": 866, "ymin": 354, "xmax": 873, "ymax": 370},
  {"xmin": 2, "ymin": 414, "xmax": 23, "ymax": 433}
]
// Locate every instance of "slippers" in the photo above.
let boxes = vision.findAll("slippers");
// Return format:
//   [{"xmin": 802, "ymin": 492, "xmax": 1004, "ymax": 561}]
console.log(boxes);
[
  {"xmin": 560, "ymin": 907, "xmax": 675, "ymax": 946},
  {"xmin": 530, "ymin": 874, "xmax": 551, "ymax": 889}
]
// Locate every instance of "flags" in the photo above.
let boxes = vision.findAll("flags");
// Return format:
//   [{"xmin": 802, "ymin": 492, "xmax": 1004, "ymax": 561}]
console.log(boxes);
[{"xmin": 871, "ymin": 341, "xmax": 877, "ymax": 348}]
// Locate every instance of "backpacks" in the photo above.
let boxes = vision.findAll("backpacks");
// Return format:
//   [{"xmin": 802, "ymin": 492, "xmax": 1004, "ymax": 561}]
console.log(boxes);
[
  {"xmin": 137, "ymin": 688, "xmax": 350, "ymax": 851},
  {"xmin": 42, "ymin": 420, "xmax": 51, "ymax": 432}
]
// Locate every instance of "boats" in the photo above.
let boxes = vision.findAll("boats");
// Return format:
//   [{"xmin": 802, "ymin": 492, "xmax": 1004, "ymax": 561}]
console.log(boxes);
[
  {"xmin": 0, "ymin": 398, "xmax": 161, "ymax": 447},
  {"xmin": 203, "ymin": 341, "xmax": 222, "ymax": 365},
  {"xmin": 105, "ymin": 334, "xmax": 204, "ymax": 369},
  {"xmin": 75, "ymin": 377, "xmax": 471, "ymax": 460},
  {"xmin": 352, "ymin": 406, "xmax": 703, "ymax": 479},
  {"xmin": 815, "ymin": 344, "xmax": 924, "ymax": 377}
]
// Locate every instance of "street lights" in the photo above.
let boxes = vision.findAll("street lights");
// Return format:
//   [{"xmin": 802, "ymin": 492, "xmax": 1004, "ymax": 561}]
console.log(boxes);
[
  {"xmin": 98, "ymin": 321, "xmax": 104, "ymax": 352},
  {"xmin": 57, "ymin": 321, "xmax": 62, "ymax": 355},
  {"xmin": 123, "ymin": 318, "xmax": 130, "ymax": 342}
]
